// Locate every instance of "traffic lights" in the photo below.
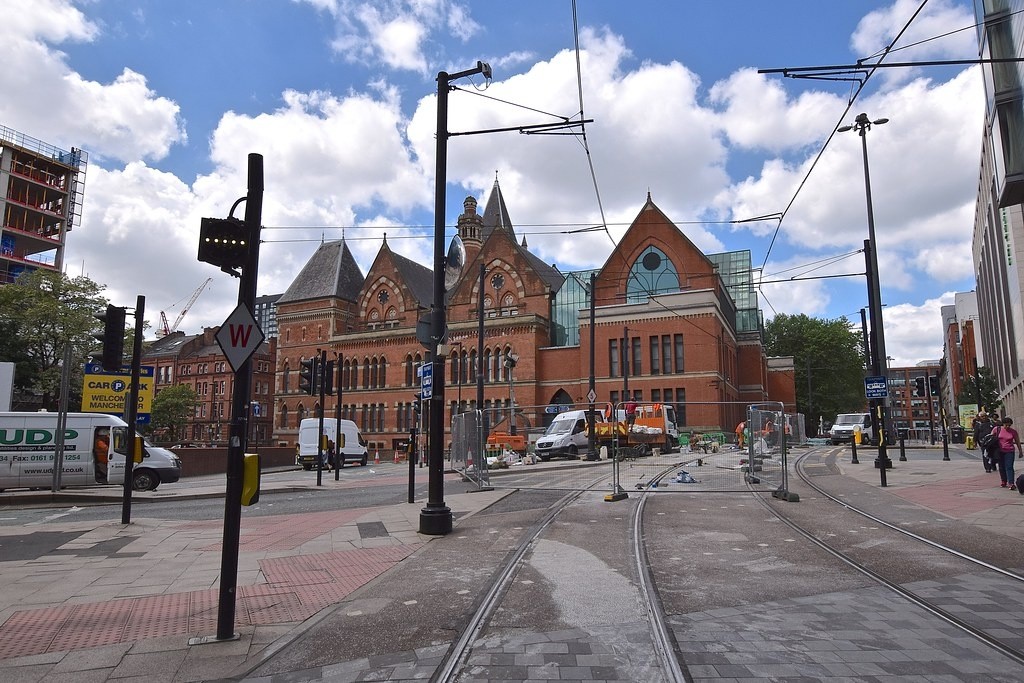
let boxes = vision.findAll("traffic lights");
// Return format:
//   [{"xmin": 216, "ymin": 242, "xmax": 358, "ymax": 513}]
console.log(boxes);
[
  {"xmin": 403, "ymin": 446, "xmax": 408, "ymax": 452},
  {"xmin": 914, "ymin": 378, "xmax": 925, "ymax": 397},
  {"xmin": 412, "ymin": 393, "xmax": 420, "ymax": 412},
  {"xmin": 299, "ymin": 359, "xmax": 312, "ymax": 394},
  {"xmin": 196, "ymin": 216, "xmax": 252, "ymax": 267},
  {"xmin": 89, "ymin": 306, "xmax": 126, "ymax": 372},
  {"xmin": 930, "ymin": 376, "xmax": 940, "ymax": 396},
  {"xmin": 409, "ymin": 428, "xmax": 415, "ymax": 444}
]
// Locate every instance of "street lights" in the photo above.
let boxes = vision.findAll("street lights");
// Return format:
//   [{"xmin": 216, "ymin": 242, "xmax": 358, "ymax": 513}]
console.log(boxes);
[{"xmin": 837, "ymin": 112, "xmax": 891, "ymax": 468}]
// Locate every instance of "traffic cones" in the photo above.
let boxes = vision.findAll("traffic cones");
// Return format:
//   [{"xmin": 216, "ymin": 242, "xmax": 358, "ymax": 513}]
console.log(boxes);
[
  {"xmin": 393, "ymin": 448, "xmax": 400, "ymax": 464},
  {"xmin": 466, "ymin": 446, "xmax": 478, "ymax": 472},
  {"xmin": 374, "ymin": 448, "xmax": 380, "ymax": 464},
  {"xmin": 352, "ymin": 461, "xmax": 360, "ymax": 467}
]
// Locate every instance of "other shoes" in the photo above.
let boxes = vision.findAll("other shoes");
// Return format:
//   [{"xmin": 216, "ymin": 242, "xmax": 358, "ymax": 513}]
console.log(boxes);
[
  {"xmin": 1007, "ymin": 483, "xmax": 1016, "ymax": 490},
  {"xmin": 986, "ymin": 469, "xmax": 991, "ymax": 473},
  {"xmin": 991, "ymin": 464, "xmax": 997, "ymax": 471},
  {"xmin": 1000, "ymin": 481, "xmax": 1007, "ymax": 488}
]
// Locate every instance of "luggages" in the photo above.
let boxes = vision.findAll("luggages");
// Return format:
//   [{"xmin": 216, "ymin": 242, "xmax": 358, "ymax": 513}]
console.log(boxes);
[{"xmin": 966, "ymin": 428, "xmax": 974, "ymax": 450}]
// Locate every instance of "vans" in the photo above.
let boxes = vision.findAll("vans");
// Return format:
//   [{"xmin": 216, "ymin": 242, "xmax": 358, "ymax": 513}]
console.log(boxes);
[
  {"xmin": 294, "ymin": 417, "xmax": 369, "ymax": 471},
  {"xmin": 535, "ymin": 408, "xmax": 626, "ymax": 460},
  {"xmin": 0, "ymin": 410, "xmax": 183, "ymax": 493}
]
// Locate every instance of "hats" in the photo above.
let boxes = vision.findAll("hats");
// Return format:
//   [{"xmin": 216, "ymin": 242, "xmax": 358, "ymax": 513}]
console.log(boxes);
[{"xmin": 979, "ymin": 411, "xmax": 987, "ymax": 416}]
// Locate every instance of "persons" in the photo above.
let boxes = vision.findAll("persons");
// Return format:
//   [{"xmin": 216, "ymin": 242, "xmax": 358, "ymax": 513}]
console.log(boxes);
[
  {"xmin": 625, "ymin": 397, "xmax": 639, "ymax": 431},
  {"xmin": 990, "ymin": 417, "xmax": 1023, "ymax": 490},
  {"xmin": 327, "ymin": 440, "xmax": 335, "ymax": 473},
  {"xmin": 95, "ymin": 430, "xmax": 110, "ymax": 464},
  {"xmin": 971, "ymin": 411, "xmax": 1001, "ymax": 472},
  {"xmin": 604, "ymin": 400, "xmax": 615, "ymax": 422},
  {"xmin": 766, "ymin": 417, "xmax": 774, "ymax": 447},
  {"xmin": 735, "ymin": 421, "xmax": 747, "ymax": 450},
  {"xmin": 785, "ymin": 422, "xmax": 790, "ymax": 441}
]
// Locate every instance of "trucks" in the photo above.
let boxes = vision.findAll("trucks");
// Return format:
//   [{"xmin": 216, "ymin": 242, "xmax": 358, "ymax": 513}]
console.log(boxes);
[
  {"xmin": 584, "ymin": 401, "xmax": 683, "ymax": 457},
  {"xmin": 830, "ymin": 412, "xmax": 872, "ymax": 446}
]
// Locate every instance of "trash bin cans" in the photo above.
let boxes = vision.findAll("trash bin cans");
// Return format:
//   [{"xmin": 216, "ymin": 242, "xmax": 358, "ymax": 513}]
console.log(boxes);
[{"xmin": 951, "ymin": 424, "xmax": 966, "ymax": 444}]
